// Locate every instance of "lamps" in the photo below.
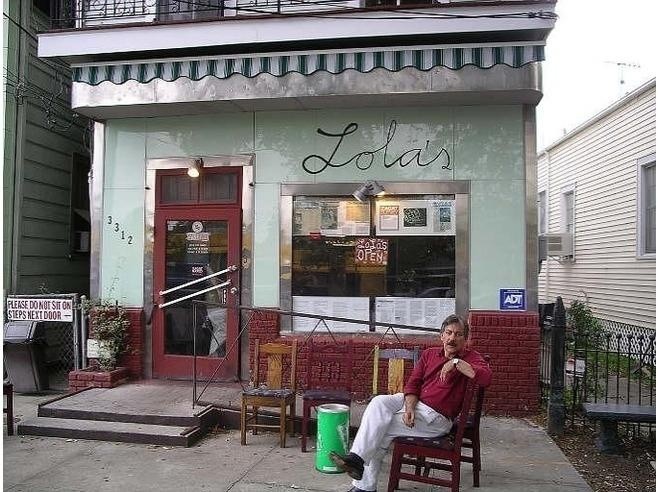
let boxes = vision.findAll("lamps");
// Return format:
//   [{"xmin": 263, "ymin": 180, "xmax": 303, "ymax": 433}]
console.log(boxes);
[
  {"xmin": 187, "ymin": 157, "xmax": 203, "ymax": 177},
  {"xmin": 350, "ymin": 181, "xmax": 385, "ymax": 203}
]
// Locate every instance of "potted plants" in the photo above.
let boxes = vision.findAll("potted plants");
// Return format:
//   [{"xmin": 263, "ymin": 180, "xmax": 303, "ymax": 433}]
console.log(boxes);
[{"xmin": 39, "ymin": 260, "xmax": 136, "ymax": 392}]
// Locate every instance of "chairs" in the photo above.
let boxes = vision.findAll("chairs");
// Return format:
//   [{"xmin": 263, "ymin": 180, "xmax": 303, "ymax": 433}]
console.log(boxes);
[
  {"xmin": 372, "ymin": 343, "xmax": 419, "ymax": 401},
  {"xmin": 240, "ymin": 338, "xmax": 297, "ymax": 448},
  {"xmin": 387, "ymin": 375, "xmax": 476, "ymax": 491},
  {"xmin": 451, "ymin": 353, "xmax": 491, "ymax": 486},
  {"xmin": 300, "ymin": 339, "xmax": 354, "ymax": 453}
]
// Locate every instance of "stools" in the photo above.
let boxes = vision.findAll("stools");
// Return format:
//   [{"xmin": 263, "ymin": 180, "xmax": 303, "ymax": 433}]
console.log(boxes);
[{"xmin": 3, "ymin": 382, "xmax": 15, "ymax": 438}]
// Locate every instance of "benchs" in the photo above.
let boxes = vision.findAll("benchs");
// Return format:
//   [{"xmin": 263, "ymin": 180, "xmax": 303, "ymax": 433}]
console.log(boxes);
[{"xmin": 580, "ymin": 400, "xmax": 655, "ymax": 452}]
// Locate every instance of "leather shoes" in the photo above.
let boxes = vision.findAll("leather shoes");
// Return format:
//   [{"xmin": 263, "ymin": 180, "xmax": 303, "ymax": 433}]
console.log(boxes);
[{"xmin": 329, "ymin": 451, "xmax": 364, "ymax": 480}]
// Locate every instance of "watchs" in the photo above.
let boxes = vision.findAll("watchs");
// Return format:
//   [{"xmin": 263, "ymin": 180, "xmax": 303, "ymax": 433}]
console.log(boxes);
[{"xmin": 452, "ymin": 358, "xmax": 460, "ymax": 370}]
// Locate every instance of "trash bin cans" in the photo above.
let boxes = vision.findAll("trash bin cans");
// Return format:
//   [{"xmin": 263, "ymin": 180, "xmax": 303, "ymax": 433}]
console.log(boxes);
[{"xmin": 3, "ymin": 320, "xmax": 50, "ymax": 395}]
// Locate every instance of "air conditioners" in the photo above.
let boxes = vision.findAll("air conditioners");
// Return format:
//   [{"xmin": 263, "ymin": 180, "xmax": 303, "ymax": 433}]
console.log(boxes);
[{"xmin": 540, "ymin": 231, "xmax": 573, "ymax": 258}]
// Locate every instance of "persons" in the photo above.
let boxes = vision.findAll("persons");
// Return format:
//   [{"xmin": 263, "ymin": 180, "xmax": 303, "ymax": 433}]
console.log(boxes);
[
  {"xmin": 330, "ymin": 314, "xmax": 492, "ymax": 491},
  {"xmin": 204, "ymin": 260, "xmax": 228, "ymax": 357}
]
